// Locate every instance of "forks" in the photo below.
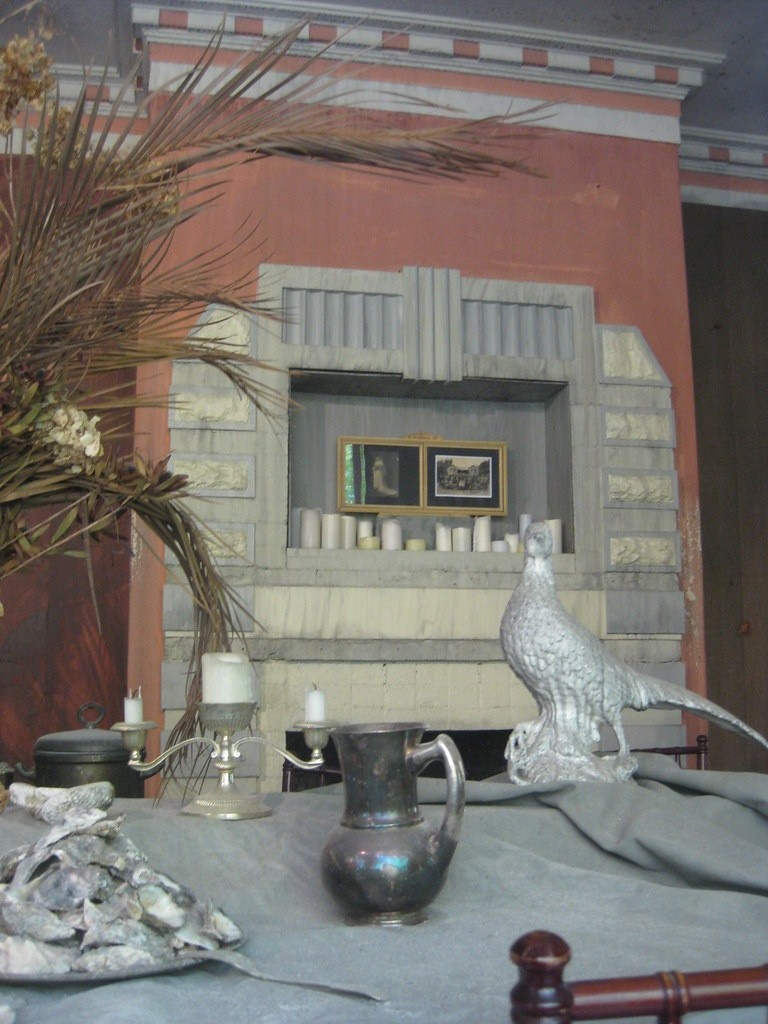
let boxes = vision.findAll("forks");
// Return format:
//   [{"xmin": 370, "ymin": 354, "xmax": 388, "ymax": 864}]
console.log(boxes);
[{"xmin": 183, "ymin": 948, "xmax": 389, "ymax": 1002}]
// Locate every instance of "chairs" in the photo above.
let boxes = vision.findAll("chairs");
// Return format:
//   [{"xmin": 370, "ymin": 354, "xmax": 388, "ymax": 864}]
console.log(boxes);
[{"xmin": 600, "ymin": 735, "xmax": 708, "ymax": 771}]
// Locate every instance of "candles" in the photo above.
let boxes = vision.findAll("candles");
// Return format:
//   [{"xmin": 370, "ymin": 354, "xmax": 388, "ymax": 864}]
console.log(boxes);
[
  {"xmin": 290, "ymin": 505, "xmax": 562, "ymax": 553},
  {"xmin": 123, "ymin": 686, "xmax": 143, "ymax": 724},
  {"xmin": 201, "ymin": 651, "xmax": 252, "ymax": 703},
  {"xmin": 306, "ymin": 682, "xmax": 325, "ymax": 723}
]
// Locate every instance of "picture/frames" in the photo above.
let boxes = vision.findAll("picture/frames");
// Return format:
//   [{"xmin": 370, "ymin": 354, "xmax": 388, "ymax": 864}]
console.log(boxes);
[{"xmin": 335, "ymin": 436, "xmax": 508, "ymax": 517}]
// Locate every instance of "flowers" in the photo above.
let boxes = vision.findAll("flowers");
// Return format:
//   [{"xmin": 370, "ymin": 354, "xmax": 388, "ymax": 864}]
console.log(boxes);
[{"xmin": 36, "ymin": 403, "xmax": 102, "ymax": 466}]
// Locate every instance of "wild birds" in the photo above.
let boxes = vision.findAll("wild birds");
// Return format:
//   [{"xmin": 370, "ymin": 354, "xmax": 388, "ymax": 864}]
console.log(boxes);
[{"xmin": 499, "ymin": 520, "xmax": 767, "ymax": 763}]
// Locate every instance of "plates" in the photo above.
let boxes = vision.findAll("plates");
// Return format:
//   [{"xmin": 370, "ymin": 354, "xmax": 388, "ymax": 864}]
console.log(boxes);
[{"xmin": 0, "ymin": 917, "xmax": 247, "ymax": 986}]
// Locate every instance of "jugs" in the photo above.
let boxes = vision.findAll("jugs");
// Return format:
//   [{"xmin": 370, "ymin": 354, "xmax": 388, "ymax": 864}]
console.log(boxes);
[{"xmin": 320, "ymin": 720, "xmax": 468, "ymax": 927}]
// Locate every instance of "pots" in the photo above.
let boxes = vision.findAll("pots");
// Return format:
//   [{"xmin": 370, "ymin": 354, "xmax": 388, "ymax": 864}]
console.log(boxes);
[{"xmin": 18, "ymin": 700, "xmax": 148, "ymax": 799}]
